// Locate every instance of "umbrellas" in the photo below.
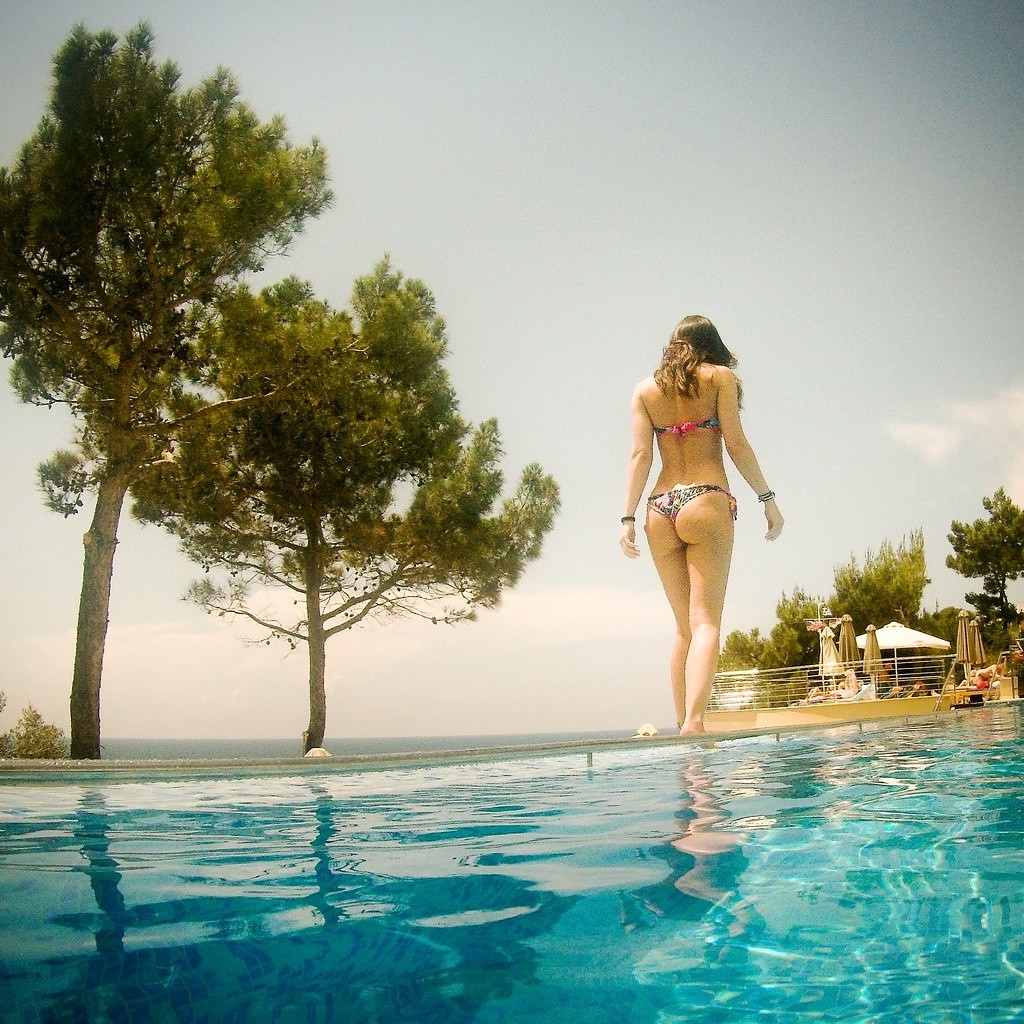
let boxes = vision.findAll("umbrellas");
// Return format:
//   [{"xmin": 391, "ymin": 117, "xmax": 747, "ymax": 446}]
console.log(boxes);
[{"xmin": 818, "ymin": 610, "xmax": 986, "ymax": 701}]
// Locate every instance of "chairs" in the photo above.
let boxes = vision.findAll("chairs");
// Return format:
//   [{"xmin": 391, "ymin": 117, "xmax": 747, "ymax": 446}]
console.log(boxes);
[{"xmin": 799, "ymin": 681, "xmax": 997, "ymax": 702}]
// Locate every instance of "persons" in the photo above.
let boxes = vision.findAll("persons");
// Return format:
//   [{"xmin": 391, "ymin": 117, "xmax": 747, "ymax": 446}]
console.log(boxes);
[
  {"xmin": 620, "ymin": 314, "xmax": 785, "ymax": 735},
  {"xmin": 799, "ymin": 657, "xmax": 1016, "ymax": 706}
]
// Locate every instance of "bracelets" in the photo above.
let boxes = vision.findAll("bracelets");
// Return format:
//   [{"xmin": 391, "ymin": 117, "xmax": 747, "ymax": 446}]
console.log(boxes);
[
  {"xmin": 621, "ymin": 515, "xmax": 636, "ymax": 523},
  {"xmin": 758, "ymin": 491, "xmax": 777, "ymax": 503}
]
